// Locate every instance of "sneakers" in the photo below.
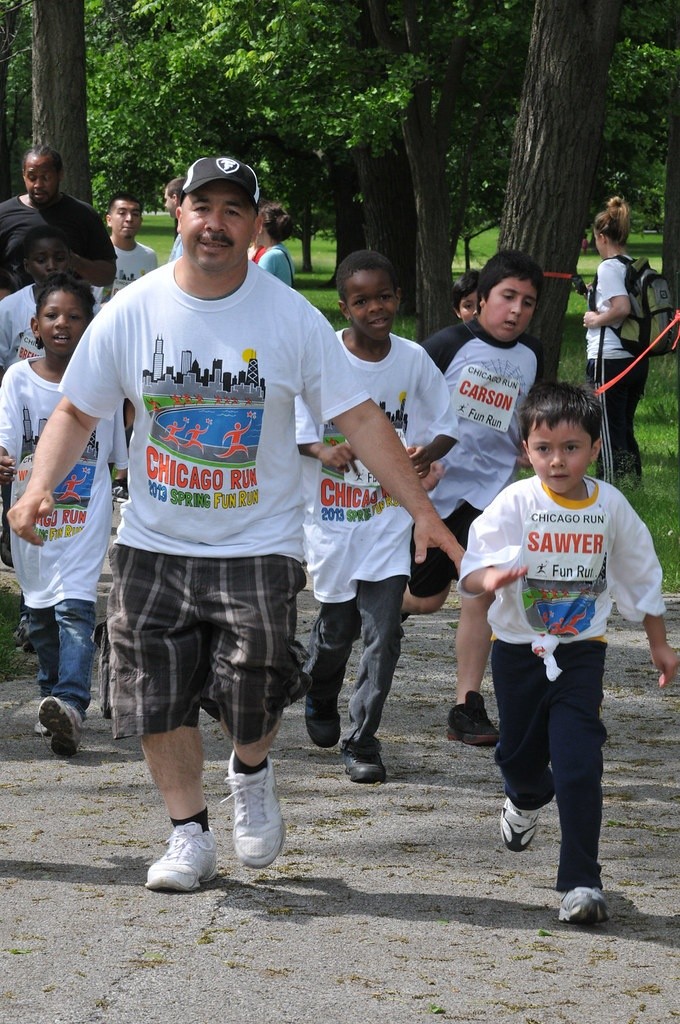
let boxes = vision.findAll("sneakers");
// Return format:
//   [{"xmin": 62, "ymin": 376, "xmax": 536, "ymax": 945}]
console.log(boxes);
[
  {"xmin": 500, "ymin": 797, "xmax": 539, "ymax": 852},
  {"xmin": 448, "ymin": 691, "xmax": 499, "ymax": 744},
  {"xmin": 220, "ymin": 748, "xmax": 286, "ymax": 869},
  {"xmin": 401, "ymin": 613, "xmax": 410, "ymax": 623},
  {"xmin": 342, "ymin": 749, "xmax": 386, "ymax": 782},
  {"xmin": 13, "ymin": 619, "xmax": 36, "ymax": 654},
  {"xmin": 34, "ymin": 696, "xmax": 83, "ymax": 756},
  {"xmin": 559, "ymin": 887, "xmax": 610, "ymax": 923},
  {"xmin": 305, "ymin": 695, "xmax": 341, "ymax": 748},
  {"xmin": 144, "ymin": 822, "xmax": 218, "ymax": 892}
]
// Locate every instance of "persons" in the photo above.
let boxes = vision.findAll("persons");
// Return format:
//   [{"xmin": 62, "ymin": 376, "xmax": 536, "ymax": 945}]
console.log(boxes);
[
  {"xmin": 575, "ymin": 194, "xmax": 651, "ymax": 508},
  {"xmin": 454, "ymin": 380, "xmax": 680, "ymax": 927},
  {"xmin": 0, "ymin": 143, "xmax": 546, "ymax": 756},
  {"xmin": 5, "ymin": 154, "xmax": 467, "ymax": 894},
  {"xmin": 295, "ymin": 248, "xmax": 463, "ymax": 786}
]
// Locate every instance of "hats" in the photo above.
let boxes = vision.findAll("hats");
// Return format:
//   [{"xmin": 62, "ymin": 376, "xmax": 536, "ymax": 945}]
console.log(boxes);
[{"xmin": 180, "ymin": 157, "xmax": 260, "ymax": 216}]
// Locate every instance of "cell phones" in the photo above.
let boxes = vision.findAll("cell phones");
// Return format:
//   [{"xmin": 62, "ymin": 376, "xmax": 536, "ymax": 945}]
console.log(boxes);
[{"xmin": 571, "ymin": 274, "xmax": 589, "ymax": 294}]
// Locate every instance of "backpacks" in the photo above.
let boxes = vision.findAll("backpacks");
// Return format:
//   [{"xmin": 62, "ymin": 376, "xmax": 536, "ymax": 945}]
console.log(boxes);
[{"xmin": 589, "ymin": 255, "xmax": 677, "ymax": 357}]
